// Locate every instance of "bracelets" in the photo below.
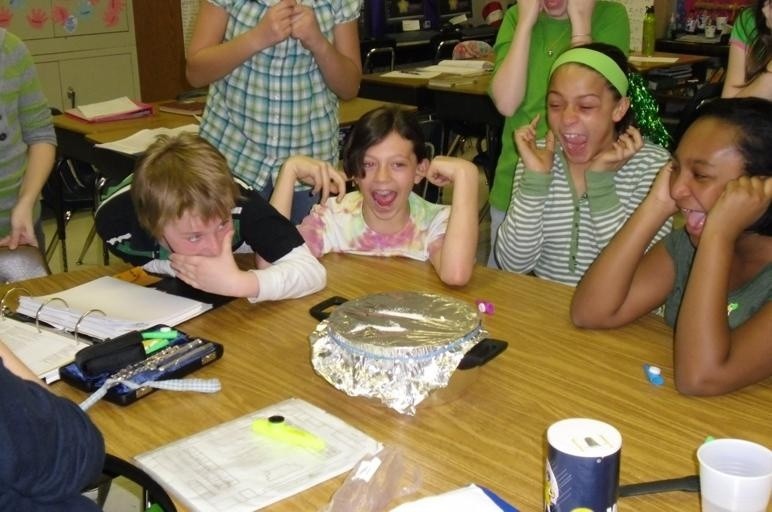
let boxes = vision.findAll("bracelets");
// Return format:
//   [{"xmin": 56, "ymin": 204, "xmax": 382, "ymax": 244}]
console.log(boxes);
[{"xmin": 573, "ymin": 34, "xmax": 591, "ymax": 36}]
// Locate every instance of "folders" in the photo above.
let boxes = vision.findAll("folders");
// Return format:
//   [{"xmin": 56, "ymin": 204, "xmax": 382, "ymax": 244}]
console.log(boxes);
[{"xmin": 65, "ymin": 99, "xmax": 155, "ymax": 123}]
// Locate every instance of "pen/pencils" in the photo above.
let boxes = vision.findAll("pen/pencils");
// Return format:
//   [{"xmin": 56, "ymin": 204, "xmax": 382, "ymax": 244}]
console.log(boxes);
[{"xmin": 136, "ymin": 328, "xmax": 216, "ymax": 372}]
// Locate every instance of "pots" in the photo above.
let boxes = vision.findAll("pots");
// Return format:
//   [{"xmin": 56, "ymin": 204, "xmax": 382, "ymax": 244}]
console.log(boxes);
[{"xmin": 309, "ymin": 293, "xmax": 507, "ymax": 410}]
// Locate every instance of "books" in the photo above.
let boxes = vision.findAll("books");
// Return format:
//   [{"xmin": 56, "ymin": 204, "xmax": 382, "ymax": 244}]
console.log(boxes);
[
  {"xmin": 419, "ymin": 60, "xmax": 494, "ymax": 78},
  {"xmin": 656, "ymin": 63, "xmax": 693, "ymax": 79},
  {"xmin": 1, "ymin": 276, "xmax": 214, "ymax": 380},
  {"xmin": 159, "ymin": 99, "xmax": 206, "ymax": 115}
]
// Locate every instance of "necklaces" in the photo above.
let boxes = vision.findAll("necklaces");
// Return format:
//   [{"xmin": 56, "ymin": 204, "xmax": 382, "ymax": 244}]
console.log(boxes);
[{"xmin": 541, "ymin": 18, "xmax": 570, "ymax": 55}]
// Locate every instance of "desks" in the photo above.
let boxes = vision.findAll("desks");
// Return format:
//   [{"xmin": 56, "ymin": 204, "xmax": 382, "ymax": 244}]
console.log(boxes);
[
  {"xmin": 0, "ymin": 253, "xmax": 771, "ymax": 512},
  {"xmin": 46, "ymin": 81, "xmax": 422, "ymax": 276},
  {"xmin": 358, "ymin": 32, "xmax": 711, "ymax": 206}
]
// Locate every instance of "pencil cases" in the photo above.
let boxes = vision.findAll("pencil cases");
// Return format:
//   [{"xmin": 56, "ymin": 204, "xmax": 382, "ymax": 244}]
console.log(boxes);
[{"xmin": 59, "ymin": 324, "xmax": 224, "ymax": 406}]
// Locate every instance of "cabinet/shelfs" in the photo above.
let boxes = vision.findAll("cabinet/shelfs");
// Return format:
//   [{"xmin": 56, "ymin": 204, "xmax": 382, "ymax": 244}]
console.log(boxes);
[{"xmin": 1, "ymin": 1, "xmax": 142, "ymax": 115}]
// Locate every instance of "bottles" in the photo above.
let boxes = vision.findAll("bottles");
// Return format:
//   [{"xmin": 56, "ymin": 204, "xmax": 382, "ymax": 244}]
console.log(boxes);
[{"xmin": 642, "ymin": 7, "xmax": 655, "ymax": 57}]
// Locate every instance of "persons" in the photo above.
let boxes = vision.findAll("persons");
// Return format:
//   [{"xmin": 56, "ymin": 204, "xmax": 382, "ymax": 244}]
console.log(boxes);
[
  {"xmin": 188, "ymin": 0, "xmax": 360, "ymax": 225},
  {"xmin": 494, "ymin": 43, "xmax": 672, "ymax": 319},
  {"xmin": 270, "ymin": 106, "xmax": 479, "ymax": 286},
  {"xmin": 1, "ymin": 28, "xmax": 57, "ymax": 255},
  {"xmin": 486, "ymin": 2, "xmax": 631, "ymax": 268},
  {"xmin": 570, "ymin": 98, "xmax": 772, "ymax": 395},
  {"xmin": 93, "ymin": 131, "xmax": 327, "ymax": 305},
  {"xmin": 1, "ymin": 356, "xmax": 104, "ymax": 511},
  {"xmin": 721, "ymin": 0, "xmax": 770, "ymax": 99}
]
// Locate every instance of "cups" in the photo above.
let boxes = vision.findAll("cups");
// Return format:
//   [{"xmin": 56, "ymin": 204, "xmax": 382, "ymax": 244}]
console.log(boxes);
[
  {"xmin": 694, "ymin": 437, "xmax": 772, "ymax": 512},
  {"xmin": 668, "ymin": 14, "xmax": 728, "ymax": 40}
]
